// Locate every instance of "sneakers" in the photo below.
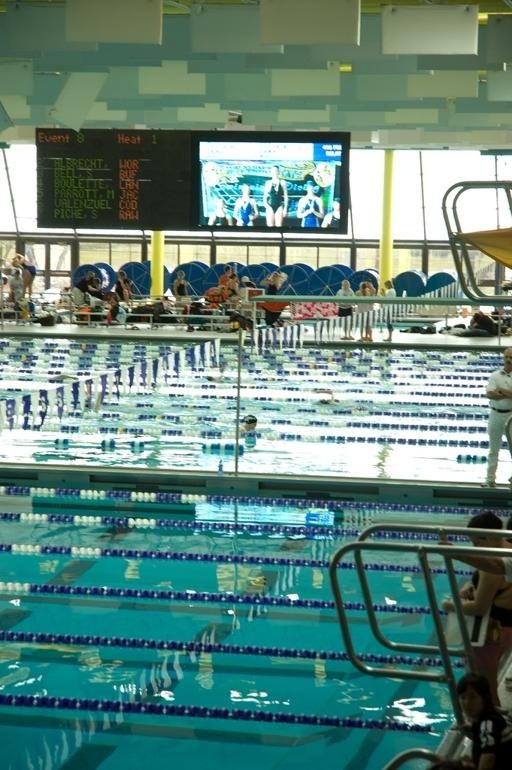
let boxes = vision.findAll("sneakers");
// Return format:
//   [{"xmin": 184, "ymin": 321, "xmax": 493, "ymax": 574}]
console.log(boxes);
[{"xmin": 481, "ymin": 482, "xmax": 496, "ymax": 488}]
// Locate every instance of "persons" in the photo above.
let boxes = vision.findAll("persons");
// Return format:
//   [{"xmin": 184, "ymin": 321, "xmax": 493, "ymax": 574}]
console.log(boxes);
[
  {"xmin": 354, "ymin": 281, "xmax": 372, "ymax": 341},
  {"xmin": 102, "ymin": 292, "xmax": 121, "ymax": 328},
  {"xmin": 11, "ymin": 253, "xmax": 36, "ymax": 301},
  {"xmin": 334, "ymin": 279, "xmax": 354, "ymax": 340},
  {"xmin": 296, "ymin": 179, "xmax": 325, "ymax": 228},
  {"xmin": 225, "ymin": 273, "xmax": 240, "ymax": 298},
  {"xmin": 423, "ymin": 669, "xmax": 512, "ymax": 770},
  {"xmin": 75, "ymin": 271, "xmax": 96, "ymax": 294},
  {"xmin": 1, "ymin": 272, "xmax": 10, "ymax": 304},
  {"xmin": 218, "ymin": 263, "xmax": 240, "ymax": 287},
  {"xmin": 207, "ymin": 197, "xmax": 234, "ymax": 227},
  {"xmin": 59, "ymin": 286, "xmax": 72, "ymax": 304},
  {"xmin": 173, "ymin": 269, "xmax": 192, "ymax": 324},
  {"xmin": 85, "ymin": 277, "xmax": 104, "ymax": 300},
  {"xmin": 11, "ymin": 265, "xmax": 26, "ymax": 301},
  {"xmin": 238, "ymin": 275, "xmax": 258, "ymax": 289},
  {"xmin": 234, "ymin": 182, "xmax": 259, "ymax": 227},
  {"xmin": 366, "ymin": 278, "xmax": 377, "ymax": 341},
  {"xmin": 440, "ymin": 510, "xmax": 512, "ymax": 708},
  {"xmin": 469, "ymin": 310, "xmax": 512, "ymax": 336},
  {"xmin": 231, "ymin": 414, "xmax": 259, "ymax": 433},
  {"xmin": 318, "ymin": 197, "xmax": 342, "ymax": 228},
  {"xmin": 115, "ymin": 268, "xmax": 132, "ymax": 306},
  {"xmin": 259, "ymin": 271, "xmax": 289, "ymax": 331},
  {"xmin": 263, "ymin": 164, "xmax": 289, "ymax": 228},
  {"xmin": 480, "ymin": 348, "xmax": 512, "ymax": 488},
  {"xmin": 380, "ymin": 279, "xmax": 397, "ymax": 341}
]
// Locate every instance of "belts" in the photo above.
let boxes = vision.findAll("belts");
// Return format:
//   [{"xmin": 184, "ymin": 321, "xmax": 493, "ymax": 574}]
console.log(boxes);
[{"xmin": 492, "ymin": 407, "xmax": 512, "ymax": 413}]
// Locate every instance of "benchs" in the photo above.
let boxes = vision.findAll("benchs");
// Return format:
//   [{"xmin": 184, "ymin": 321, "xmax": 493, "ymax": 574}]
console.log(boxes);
[{"xmin": 72, "ymin": 310, "xmax": 230, "ymax": 331}]
